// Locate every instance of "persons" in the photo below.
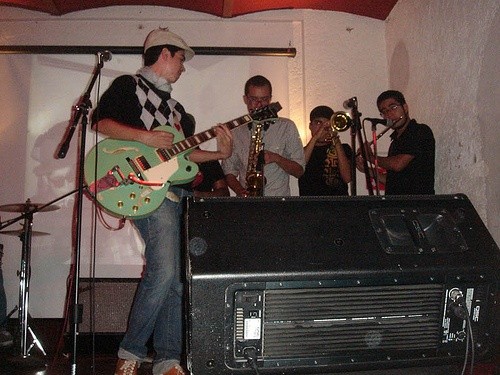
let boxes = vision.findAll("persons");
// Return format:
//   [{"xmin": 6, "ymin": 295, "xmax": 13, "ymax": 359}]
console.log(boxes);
[
  {"xmin": 186, "ymin": 113, "xmax": 230, "ymax": 197},
  {"xmin": 356, "ymin": 90, "xmax": 435, "ymax": 195},
  {"xmin": 222, "ymin": 75, "xmax": 306, "ymax": 197},
  {"xmin": 91, "ymin": 31, "xmax": 233, "ymax": 375},
  {"xmin": 298, "ymin": 106, "xmax": 353, "ymax": 196}
]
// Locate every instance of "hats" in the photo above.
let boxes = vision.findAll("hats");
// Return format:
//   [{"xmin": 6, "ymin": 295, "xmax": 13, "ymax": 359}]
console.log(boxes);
[
  {"xmin": 310, "ymin": 105, "xmax": 334, "ymax": 122},
  {"xmin": 143, "ymin": 29, "xmax": 195, "ymax": 62}
]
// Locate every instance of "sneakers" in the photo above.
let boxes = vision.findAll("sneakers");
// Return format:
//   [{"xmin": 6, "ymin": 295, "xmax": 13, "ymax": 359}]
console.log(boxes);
[
  {"xmin": 162, "ymin": 365, "xmax": 186, "ymax": 375},
  {"xmin": 114, "ymin": 357, "xmax": 139, "ymax": 375}
]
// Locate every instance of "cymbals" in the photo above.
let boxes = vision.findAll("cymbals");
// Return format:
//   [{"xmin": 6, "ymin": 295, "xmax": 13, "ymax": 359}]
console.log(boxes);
[{"xmin": 0, "ymin": 203, "xmax": 60, "ymax": 212}]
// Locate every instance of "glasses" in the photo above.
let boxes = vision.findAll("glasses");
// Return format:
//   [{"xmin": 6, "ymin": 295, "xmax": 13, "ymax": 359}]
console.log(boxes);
[
  {"xmin": 246, "ymin": 93, "xmax": 270, "ymax": 105},
  {"xmin": 313, "ymin": 119, "xmax": 324, "ymax": 126},
  {"xmin": 380, "ymin": 103, "xmax": 402, "ymax": 118}
]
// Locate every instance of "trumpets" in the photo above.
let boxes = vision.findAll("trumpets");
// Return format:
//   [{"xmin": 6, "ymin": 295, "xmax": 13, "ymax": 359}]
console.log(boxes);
[{"xmin": 325, "ymin": 111, "xmax": 353, "ymax": 132}]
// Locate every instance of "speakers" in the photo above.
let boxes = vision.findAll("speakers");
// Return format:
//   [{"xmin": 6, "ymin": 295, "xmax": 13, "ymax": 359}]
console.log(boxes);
[
  {"xmin": 68, "ymin": 277, "xmax": 156, "ymax": 356},
  {"xmin": 180, "ymin": 192, "xmax": 500, "ymax": 375}
]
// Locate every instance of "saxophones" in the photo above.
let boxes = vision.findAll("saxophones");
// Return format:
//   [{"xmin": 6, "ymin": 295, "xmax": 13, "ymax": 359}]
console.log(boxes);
[{"xmin": 245, "ymin": 122, "xmax": 267, "ymax": 195}]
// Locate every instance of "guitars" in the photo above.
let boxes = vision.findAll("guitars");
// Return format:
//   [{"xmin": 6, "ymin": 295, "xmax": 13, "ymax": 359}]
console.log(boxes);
[{"xmin": 83, "ymin": 101, "xmax": 283, "ymax": 221}]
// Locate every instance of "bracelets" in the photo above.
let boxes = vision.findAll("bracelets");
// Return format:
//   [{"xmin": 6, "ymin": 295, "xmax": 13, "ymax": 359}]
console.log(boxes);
[{"xmin": 335, "ymin": 140, "xmax": 340, "ymax": 144}]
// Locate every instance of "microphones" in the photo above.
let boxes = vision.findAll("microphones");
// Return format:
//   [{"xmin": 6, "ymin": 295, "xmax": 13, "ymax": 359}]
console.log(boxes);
[
  {"xmin": 364, "ymin": 117, "xmax": 393, "ymax": 127},
  {"xmin": 97, "ymin": 49, "xmax": 112, "ymax": 62},
  {"xmin": 343, "ymin": 96, "xmax": 356, "ymax": 110}
]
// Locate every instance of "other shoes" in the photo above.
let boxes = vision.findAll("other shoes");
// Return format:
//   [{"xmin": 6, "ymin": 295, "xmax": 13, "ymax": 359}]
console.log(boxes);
[{"xmin": 0, "ymin": 330, "xmax": 14, "ymax": 346}]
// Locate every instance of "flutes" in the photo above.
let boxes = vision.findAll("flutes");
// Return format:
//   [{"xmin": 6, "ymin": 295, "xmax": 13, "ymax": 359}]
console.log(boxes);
[{"xmin": 356, "ymin": 115, "xmax": 402, "ymax": 159}]
